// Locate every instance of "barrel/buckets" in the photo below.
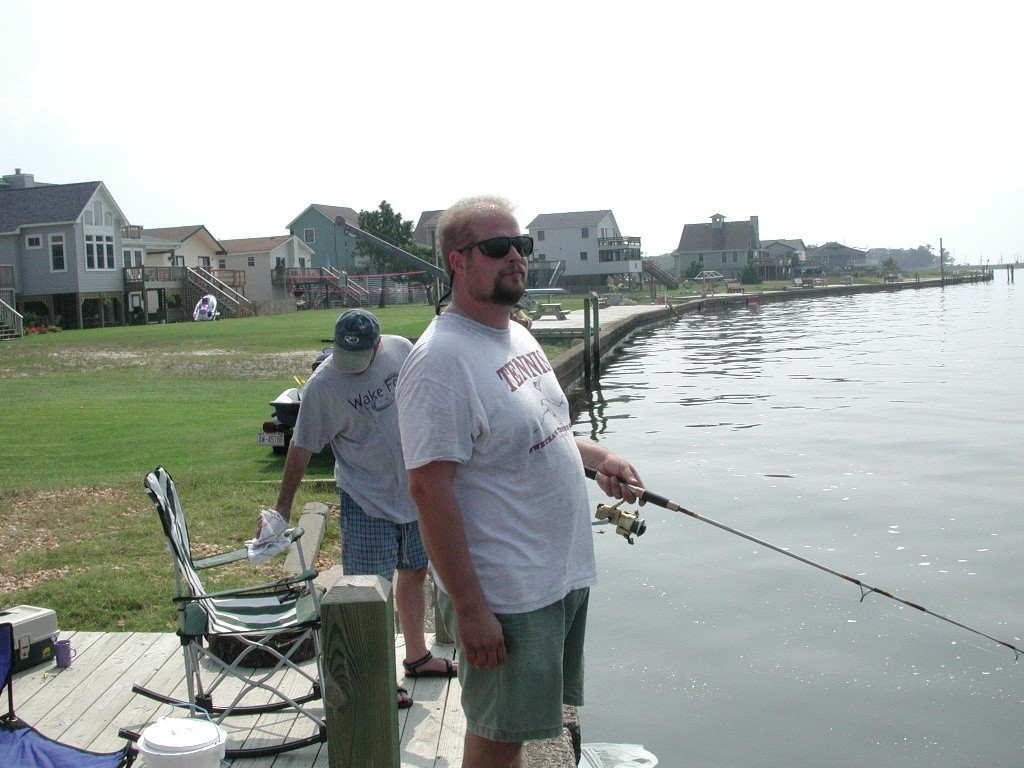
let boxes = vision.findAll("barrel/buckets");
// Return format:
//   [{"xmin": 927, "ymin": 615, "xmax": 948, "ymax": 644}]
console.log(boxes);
[{"xmin": 136, "ymin": 703, "xmax": 228, "ymax": 768}]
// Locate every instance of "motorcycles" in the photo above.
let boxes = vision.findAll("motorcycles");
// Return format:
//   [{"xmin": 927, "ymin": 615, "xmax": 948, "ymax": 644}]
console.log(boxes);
[
  {"xmin": 262, "ymin": 339, "xmax": 336, "ymax": 456},
  {"xmin": 192, "ymin": 294, "xmax": 217, "ymax": 321}
]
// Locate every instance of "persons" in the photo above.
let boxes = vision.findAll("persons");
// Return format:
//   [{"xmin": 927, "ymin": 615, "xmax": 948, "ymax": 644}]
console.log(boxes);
[
  {"xmin": 256, "ymin": 310, "xmax": 458, "ymax": 708},
  {"xmin": 394, "ymin": 196, "xmax": 646, "ymax": 768},
  {"xmin": 275, "ymin": 260, "xmax": 284, "ymax": 280},
  {"xmin": 199, "ymin": 298, "xmax": 213, "ymax": 317}
]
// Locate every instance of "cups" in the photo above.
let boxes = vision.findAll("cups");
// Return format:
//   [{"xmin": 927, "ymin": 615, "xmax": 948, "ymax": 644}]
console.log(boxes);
[{"xmin": 53, "ymin": 639, "xmax": 77, "ymax": 667}]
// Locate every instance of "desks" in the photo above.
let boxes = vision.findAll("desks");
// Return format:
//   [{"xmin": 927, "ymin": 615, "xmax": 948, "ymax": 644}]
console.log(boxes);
[{"xmin": 532, "ymin": 303, "xmax": 566, "ymax": 321}]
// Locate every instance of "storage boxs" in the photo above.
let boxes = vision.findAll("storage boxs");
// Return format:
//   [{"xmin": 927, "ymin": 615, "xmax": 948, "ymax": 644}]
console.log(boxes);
[{"xmin": 0, "ymin": 605, "xmax": 60, "ymax": 673}]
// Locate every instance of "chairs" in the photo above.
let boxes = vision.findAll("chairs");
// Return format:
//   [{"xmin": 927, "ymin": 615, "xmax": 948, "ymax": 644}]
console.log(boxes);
[
  {"xmin": 0, "ymin": 623, "xmax": 136, "ymax": 768},
  {"xmin": 119, "ymin": 466, "xmax": 327, "ymax": 756}
]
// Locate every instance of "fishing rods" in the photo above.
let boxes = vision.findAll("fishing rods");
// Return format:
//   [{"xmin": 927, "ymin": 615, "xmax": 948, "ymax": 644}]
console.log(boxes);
[{"xmin": 584, "ymin": 467, "xmax": 1024, "ymax": 658}]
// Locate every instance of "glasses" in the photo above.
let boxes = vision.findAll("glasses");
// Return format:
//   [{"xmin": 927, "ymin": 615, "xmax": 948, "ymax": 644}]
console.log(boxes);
[{"xmin": 459, "ymin": 236, "xmax": 535, "ymax": 259}]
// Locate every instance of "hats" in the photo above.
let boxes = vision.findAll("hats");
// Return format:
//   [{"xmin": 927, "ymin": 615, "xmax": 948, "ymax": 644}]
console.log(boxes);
[{"xmin": 332, "ymin": 309, "xmax": 380, "ymax": 375}]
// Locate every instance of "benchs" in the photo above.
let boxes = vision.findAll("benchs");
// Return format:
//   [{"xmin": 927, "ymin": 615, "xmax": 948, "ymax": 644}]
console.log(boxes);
[
  {"xmin": 526, "ymin": 309, "xmax": 570, "ymax": 315},
  {"xmin": 725, "ymin": 276, "xmax": 899, "ymax": 292}
]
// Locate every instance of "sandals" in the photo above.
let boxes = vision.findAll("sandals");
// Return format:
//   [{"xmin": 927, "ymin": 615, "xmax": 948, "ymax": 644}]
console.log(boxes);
[
  {"xmin": 398, "ymin": 683, "xmax": 414, "ymax": 709},
  {"xmin": 403, "ymin": 650, "xmax": 458, "ymax": 679}
]
